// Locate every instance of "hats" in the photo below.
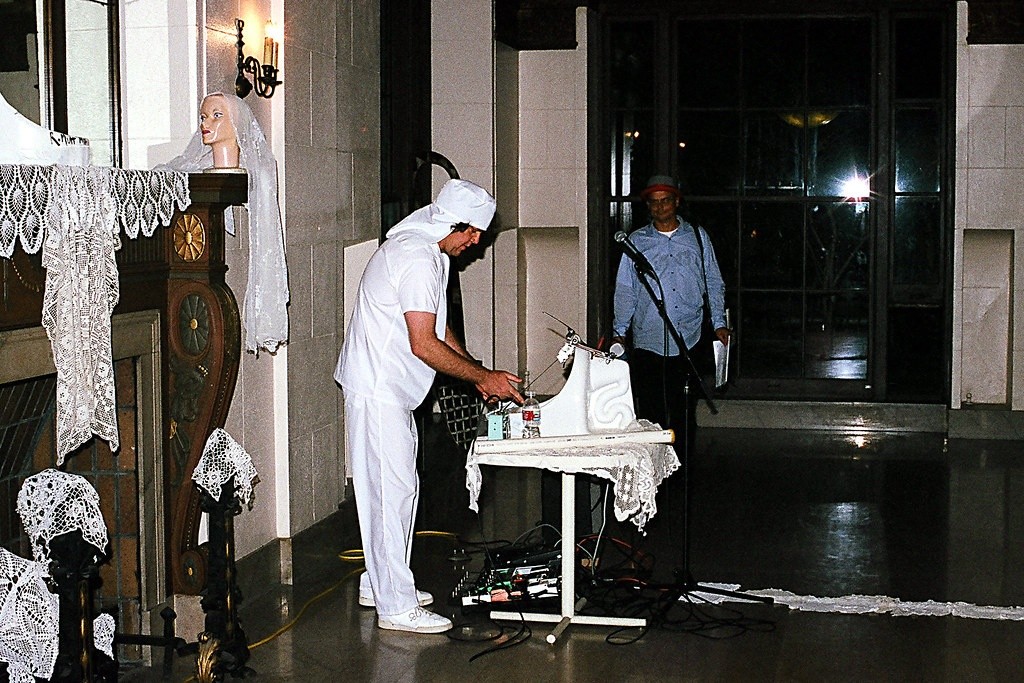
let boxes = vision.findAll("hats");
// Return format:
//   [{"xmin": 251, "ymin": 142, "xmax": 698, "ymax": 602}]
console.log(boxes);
[
  {"xmin": 641, "ymin": 175, "xmax": 679, "ymax": 196},
  {"xmin": 435, "ymin": 179, "xmax": 497, "ymax": 230}
]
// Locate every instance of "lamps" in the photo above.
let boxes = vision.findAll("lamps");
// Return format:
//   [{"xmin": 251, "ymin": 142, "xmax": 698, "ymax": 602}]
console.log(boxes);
[{"xmin": 234, "ymin": 18, "xmax": 283, "ymax": 100}]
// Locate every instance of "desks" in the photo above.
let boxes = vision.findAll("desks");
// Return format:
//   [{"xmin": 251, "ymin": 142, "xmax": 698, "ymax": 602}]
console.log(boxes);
[{"xmin": 475, "ymin": 428, "xmax": 677, "ymax": 645}]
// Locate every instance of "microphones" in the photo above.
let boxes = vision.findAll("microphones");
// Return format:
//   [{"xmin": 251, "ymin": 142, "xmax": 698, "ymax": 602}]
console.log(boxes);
[{"xmin": 614, "ymin": 230, "xmax": 659, "ymax": 281}]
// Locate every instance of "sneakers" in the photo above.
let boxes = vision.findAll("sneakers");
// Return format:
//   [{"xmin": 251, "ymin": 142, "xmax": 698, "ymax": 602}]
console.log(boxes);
[
  {"xmin": 359, "ymin": 587, "xmax": 434, "ymax": 605},
  {"xmin": 378, "ymin": 607, "xmax": 452, "ymax": 633}
]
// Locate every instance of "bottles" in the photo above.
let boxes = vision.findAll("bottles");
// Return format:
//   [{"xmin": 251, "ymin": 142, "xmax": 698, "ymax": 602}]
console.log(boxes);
[{"xmin": 522, "ymin": 391, "xmax": 541, "ymax": 438}]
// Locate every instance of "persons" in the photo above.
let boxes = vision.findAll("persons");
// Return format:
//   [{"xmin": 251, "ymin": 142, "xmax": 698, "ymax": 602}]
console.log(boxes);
[
  {"xmin": 612, "ymin": 173, "xmax": 732, "ymax": 501},
  {"xmin": 196, "ymin": 90, "xmax": 246, "ymax": 172},
  {"xmin": 331, "ymin": 176, "xmax": 526, "ymax": 637}
]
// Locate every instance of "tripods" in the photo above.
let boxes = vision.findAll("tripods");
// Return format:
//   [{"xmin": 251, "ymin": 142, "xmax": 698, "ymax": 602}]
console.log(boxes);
[{"xmin": 631, "ymin": 272, "xmax": 775, "ymax": 624}]
